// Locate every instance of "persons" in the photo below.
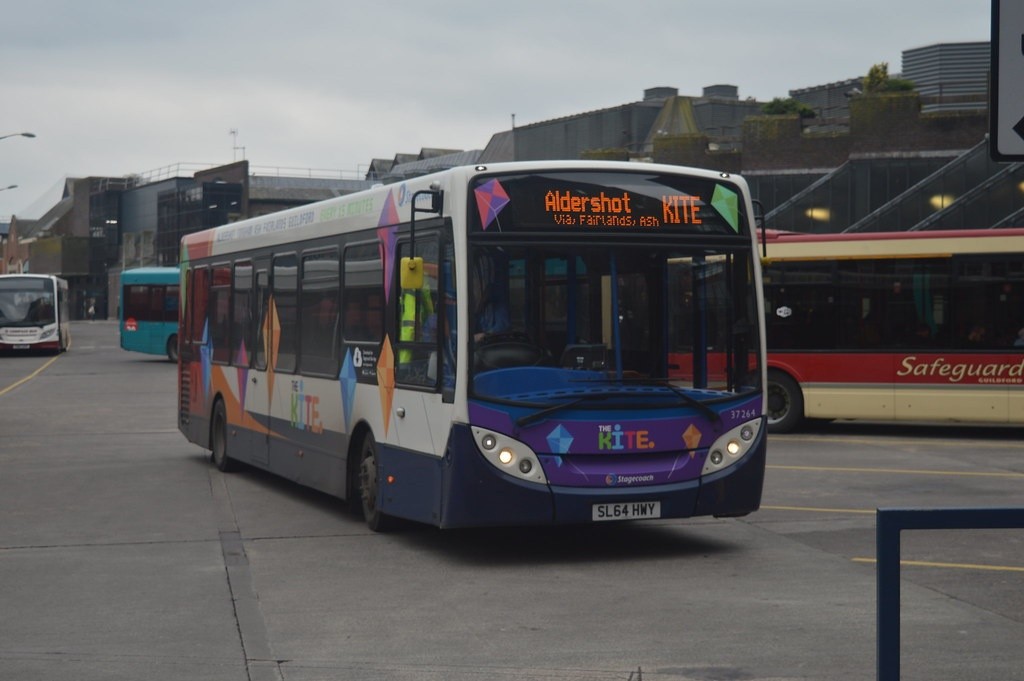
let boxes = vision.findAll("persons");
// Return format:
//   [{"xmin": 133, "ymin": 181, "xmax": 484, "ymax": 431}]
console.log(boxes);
[
  {"xmin": 473, "ymin": 283, "xmax": 510, "ymax": 341},
  {"xmin": 92, "ymin": 304, "xmax": 97, "ymax": 321}
]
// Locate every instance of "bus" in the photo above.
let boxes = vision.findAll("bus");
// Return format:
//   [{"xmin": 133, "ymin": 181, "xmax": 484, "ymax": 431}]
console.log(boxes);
[
  {"xmin": 0, "ymin": 274, "xmax": 70, "ymax": 353},
  {"xmin": 176, "ymin": 161, "xmax": 770, "ymax": 537},
  {"xmin": 233, "ymin": 259, "xmax": 437, "ymax": 384},
  {"xmin": 119, "ymin": 267, "xmax": 180, "ymax": 362},
  {"xmin": 602, "ymin": 229, "xmax": 1024, "ymax": 432}
]
